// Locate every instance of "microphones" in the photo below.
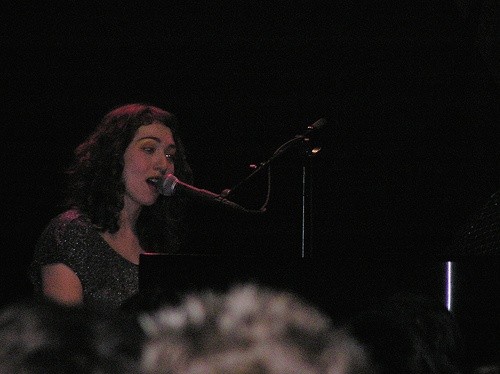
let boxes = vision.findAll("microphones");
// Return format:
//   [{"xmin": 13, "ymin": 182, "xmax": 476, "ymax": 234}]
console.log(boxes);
[{"xmin": 157, "ymin": 173, "xmax": 246, "ymax": 213}]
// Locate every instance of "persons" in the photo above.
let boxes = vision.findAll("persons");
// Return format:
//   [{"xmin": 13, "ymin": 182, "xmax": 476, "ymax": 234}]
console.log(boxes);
[
  {"xmin": 0, "ymin": 279, "xmax": 377, "ymax": 374},
  {"xmin": 23, "ymin": 105, "xmax": 195, "ymax": 330}
]
